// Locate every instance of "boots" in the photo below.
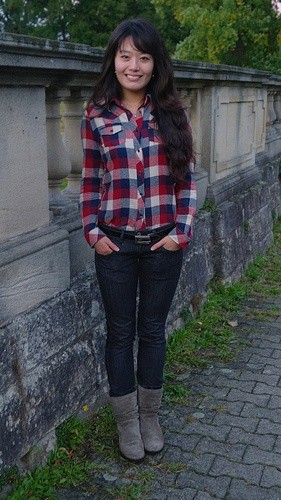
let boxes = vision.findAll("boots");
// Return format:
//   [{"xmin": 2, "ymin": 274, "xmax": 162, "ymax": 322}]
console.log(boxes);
[
  {"xmin": 137, "ymin": 383, "xmax": 164, "ymax": 455},
  {"xmin": 107, "ymin": 387, "xmax": 145, "ymax": 463}
]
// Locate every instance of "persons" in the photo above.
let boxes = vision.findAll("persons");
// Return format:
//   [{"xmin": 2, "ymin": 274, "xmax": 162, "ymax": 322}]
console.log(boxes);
[{"xmin": 78, "ymin": 18, "xmax": 199, "ymax": 463}]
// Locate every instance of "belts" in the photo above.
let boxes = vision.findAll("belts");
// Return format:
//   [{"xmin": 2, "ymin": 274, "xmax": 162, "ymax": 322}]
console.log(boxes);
[{"xmin": 97, "ymin": 223, "xmax": 177, "ymax": 245}]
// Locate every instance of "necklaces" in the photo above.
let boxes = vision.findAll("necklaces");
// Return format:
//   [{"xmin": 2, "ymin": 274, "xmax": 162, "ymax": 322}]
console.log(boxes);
[{"xmin": 122, "ymin": 102, "xmax": 146, "ymax": 118}]
